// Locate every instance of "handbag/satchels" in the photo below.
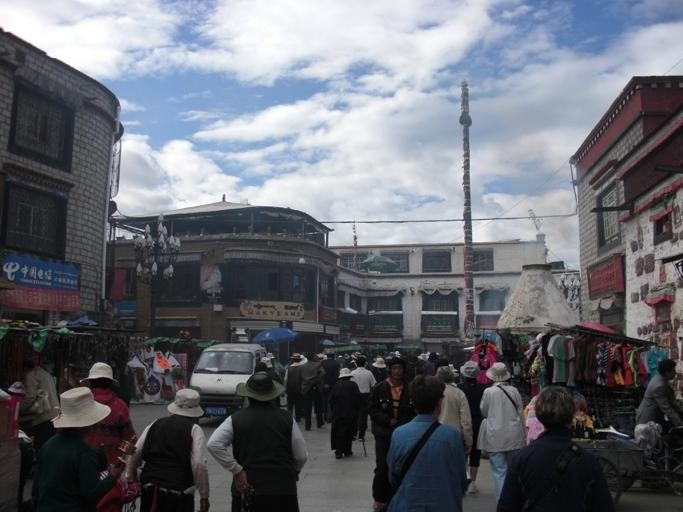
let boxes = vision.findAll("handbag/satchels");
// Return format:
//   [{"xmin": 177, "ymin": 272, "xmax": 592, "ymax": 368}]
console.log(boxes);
[{"xmin": 27, "ymin": 389, "xmax": 50, "ymax": 415}]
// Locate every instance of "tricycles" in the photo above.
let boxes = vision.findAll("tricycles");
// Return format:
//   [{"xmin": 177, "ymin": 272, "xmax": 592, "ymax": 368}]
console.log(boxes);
[{"xmin": 567, "ymin": 421, "xmax": 683, "ymax": 504}]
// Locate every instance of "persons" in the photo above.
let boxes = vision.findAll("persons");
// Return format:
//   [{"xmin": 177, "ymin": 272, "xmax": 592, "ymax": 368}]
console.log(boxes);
[{"xmin": 19, "ymin": 345, "xmax": 682, "ymax": 512}]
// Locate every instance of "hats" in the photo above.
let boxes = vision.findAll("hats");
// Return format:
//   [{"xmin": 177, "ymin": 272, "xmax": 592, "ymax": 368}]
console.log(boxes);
[
  {"xmin": 50, "ymin": 387, "xmax": 111, "ymax": 428},
  {"xmin": 289, "ymin": 353, "xmax": 301, "ymax": 358},
  {"xmin": 265, "ymin": 353, "xmax": 276, "ymax": 358},
  {"xmin": 261, "ymin": 357, "xmax": 272, "ymax": 368},
  {"xmin": 372, "ymin": 358, "xmax": 387, "ymax": 368},
  {"xmin": 338, "ymin": 368, "xmax": 353, "ymax": 378},
  {"xmin": 486, "ymin": 363, "xmax": 511, "ymax": 382},
  {"xmin": 80, "ymin": 362, "xmax": 119, "ymax": 384},
  {"xmin": 237, "ymin": 371, "xmax": 286, "ymax": 401},
  {"xmin": 460, "ymin": 361, "xmax": 481, "ymax": 378},
  {"xmin": 436, "ymin": 366, "xmax": 454, "ymax": 381},
  {"xmin": 8, "ymin": 382, "xmax": 24, "ymax": 393},
  {"xmin": 417, "ymin": 354, "xmax": 429, "ymax": 361},
  {"xmin": 168, "ymin": 389, "xmax": 204, "ymax": 418}
]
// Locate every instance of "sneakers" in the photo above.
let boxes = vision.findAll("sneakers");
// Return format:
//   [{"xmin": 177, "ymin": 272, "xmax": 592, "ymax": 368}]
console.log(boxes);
[{"xmin": 467, "ymin": 482, "xmax": 479, "ymax": 493}]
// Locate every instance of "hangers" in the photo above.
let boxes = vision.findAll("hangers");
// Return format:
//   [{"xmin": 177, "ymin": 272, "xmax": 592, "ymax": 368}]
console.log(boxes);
[{"xmin": 542, "ymin": 325, "xmax": 663, "ymax": 353}]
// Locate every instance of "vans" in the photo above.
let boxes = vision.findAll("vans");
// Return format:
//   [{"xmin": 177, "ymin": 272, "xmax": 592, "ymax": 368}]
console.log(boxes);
[{"xmin": 189, "ymin": 343, "xmax": 267, "ymax": 424}]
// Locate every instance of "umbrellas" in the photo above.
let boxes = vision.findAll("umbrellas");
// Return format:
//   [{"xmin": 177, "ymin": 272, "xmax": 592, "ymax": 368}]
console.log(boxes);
[
  {"xmin": 360, "ymin": 256, "xmax": 398, "ymax": 272},
  {"xmin": 320, "ymin": 339, "xmax": 336, "ymax": 346},
  {"xmin": 251, "ymin": 326, "xmax": 298, "ymax": 358}
]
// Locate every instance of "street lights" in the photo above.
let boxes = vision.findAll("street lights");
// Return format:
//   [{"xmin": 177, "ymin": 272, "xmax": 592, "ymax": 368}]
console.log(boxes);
[{"xmin": 131, "ymin": 212, "xmax": 181, "ymax": 339}]
